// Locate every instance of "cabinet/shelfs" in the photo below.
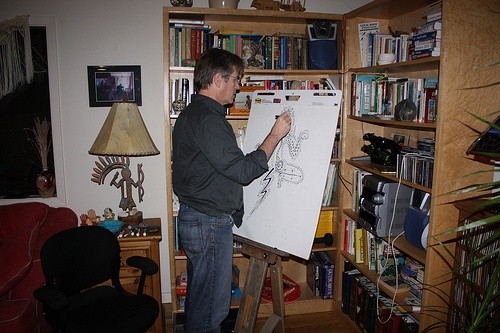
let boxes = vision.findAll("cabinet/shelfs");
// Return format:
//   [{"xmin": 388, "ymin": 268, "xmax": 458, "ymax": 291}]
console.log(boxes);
[
  {"xmin": 163, "ymin": 0, "xmax": 500, "ymax": 333},
  {"xmin": 118, "ymin": 218, "xmax": 162, "ymax": 333}
]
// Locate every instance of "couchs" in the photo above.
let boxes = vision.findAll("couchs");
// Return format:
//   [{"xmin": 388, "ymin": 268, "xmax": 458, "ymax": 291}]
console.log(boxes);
[{"xmin": 0, "ymin": 203, "xmax": 78, "ymax": 333}]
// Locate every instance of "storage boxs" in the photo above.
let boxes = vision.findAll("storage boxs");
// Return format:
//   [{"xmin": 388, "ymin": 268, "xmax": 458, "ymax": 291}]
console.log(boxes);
[
  {"xmin": 307, "ymin": 252, "xmax": 323, "ymax": 297},
  {"xmin": 317, "ymin": 252, "xmax": 336, "ymax": 300}
]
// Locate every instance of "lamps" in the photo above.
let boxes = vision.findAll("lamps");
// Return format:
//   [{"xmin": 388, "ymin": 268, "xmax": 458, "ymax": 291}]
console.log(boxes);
[{"xmin": 87, "ymin": 103, "xmax": 160, "ymax": 224}]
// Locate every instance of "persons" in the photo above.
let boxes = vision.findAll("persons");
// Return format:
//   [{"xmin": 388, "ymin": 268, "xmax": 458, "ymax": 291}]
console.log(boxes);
[
  {"xmin": 245, "ymin": 95, "xmax": 252, "ymax": 108},
  {"xmin": 172, "ymin": 47, "xmax": 292, "ymax": 333}
]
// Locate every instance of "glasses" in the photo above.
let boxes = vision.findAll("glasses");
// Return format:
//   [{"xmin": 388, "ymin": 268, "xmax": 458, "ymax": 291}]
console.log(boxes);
[{"xmin": 221, "ymin": 73, "xmax": 241, "ymax": 84}]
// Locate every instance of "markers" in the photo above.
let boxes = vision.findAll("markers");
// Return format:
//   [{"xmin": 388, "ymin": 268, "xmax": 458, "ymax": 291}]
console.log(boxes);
[
  {"xmin": 143, "ymin": 228, "xmax": 147, "ymax": 236},
  {"xmin": 117, "ymin": 227, "xmax": 140, "ymax": 238}
]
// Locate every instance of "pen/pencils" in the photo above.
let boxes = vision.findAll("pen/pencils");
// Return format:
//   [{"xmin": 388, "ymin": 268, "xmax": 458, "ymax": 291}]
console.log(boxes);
[{"xmin": 275, "ymin": 116, "xmax": 279, "ymax": 119}]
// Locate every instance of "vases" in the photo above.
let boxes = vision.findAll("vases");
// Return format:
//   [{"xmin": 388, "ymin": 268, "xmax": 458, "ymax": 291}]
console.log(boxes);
[{"xmin": 38, "ymin": 172, "xmax": 56, "ymax": 197}]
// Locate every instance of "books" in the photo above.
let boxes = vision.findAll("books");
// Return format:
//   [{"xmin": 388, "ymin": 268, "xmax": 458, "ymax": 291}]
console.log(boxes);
[
  {"xmin": 341, "ymin": 217, "xmax": 425, "ymax": 333},
  {"xmin": 305, "ymin": 252, "xmax": 336, "ymax": 300},
  {"xmin": 169, "ymin": 75, "xmax": 338, "ymax": 114},
  {"xmin": 451, "ymin": 219, "xmax": 500, "ymax": 333},
  {"xmin": 350, "ymin": 72, "xmax": 439, "ymax": 123},
  {"xmin": 357, "ymin": 0, "xmax": 443, "ymax": 68},
  {"xmin": 169, "ymin": 14, "xmax": 309, "ymax": 71},
  {"xmin": 391, "ymin": 133, "xmax": 436, "ymax": 189}
]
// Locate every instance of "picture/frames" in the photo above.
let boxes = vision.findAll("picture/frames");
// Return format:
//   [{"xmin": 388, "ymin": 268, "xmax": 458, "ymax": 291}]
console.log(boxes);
[{"xmin": 87, "ymin": 65, "xmax": 142, "ymax": 107}]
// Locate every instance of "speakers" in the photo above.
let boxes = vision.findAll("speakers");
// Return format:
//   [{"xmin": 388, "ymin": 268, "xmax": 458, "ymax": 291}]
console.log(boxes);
[
  {"xmin": 308, "ymin": 40, "xmax": 338, "ymax": 70},
  {"xmin": 404, "ymin": 209, "xmax": 430, "ymax": 251}
]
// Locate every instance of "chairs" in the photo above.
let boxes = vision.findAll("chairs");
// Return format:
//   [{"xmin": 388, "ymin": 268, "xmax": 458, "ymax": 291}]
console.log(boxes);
[{"xmin": 33, "ymin": 225, "xmax": 160, "ymax": 333}]
